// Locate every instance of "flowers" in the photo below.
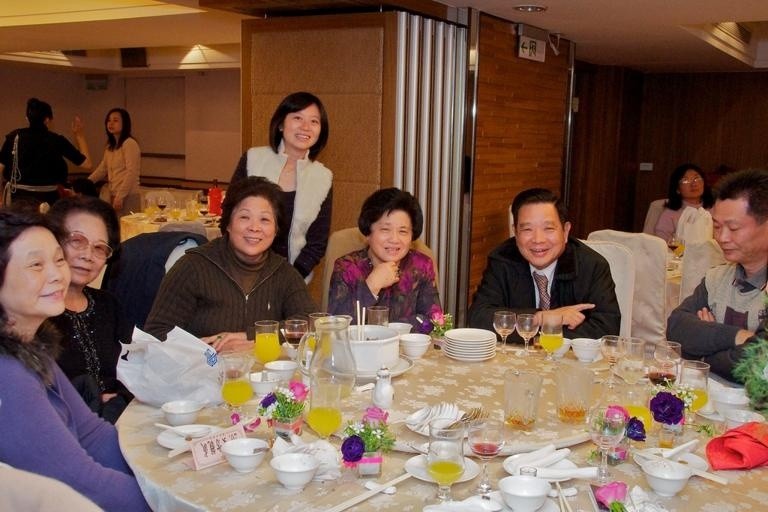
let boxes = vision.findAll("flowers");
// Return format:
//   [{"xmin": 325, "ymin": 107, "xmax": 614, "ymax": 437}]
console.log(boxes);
[
  {"xmin": 595, "ymin": 479, "xmax": 629, "ymax": 511},
  {"xmin": 427, "ymin": 303, "xmax": 454, "ymax": 339},
  {"xmin": 590, "ymin": 403, "xmax": 645, "ymax": 464},
  {"xmin": 645, "ymin": 373, "xmax": 699, "ymax": 427},
  {"xmin": 258, "ymin": 375, "xmax": 311, "ymax": 421},
  {"xmin": 336, "ymin": 405, "xmax": 395, "ymax": 469}
]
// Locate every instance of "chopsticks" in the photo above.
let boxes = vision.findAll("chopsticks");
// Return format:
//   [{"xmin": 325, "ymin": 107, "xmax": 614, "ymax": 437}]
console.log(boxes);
[
  {"xmin": 356, "ymin": 298, "xmax": 367, "ymax": 340},
  {"xmin": 330, "ymin": 473, "xmax": 412, "ymax": 510}
]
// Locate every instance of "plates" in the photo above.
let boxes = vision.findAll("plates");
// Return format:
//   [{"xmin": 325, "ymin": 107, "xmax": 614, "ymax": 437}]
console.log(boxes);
[
  {"xmin": 156, "ymin": 424, "xmax": 222, "ymax": 449},
  {"xmin": 709, "ymin": 388, "xmax": 752, "ymax": 405},
  {"xmin": 723, "ymin": 409, "xmax": 765, "ymax": 424},
  {"xmin": 504, "ymin": 452, "xmax": 578, "ymax": 480},
  {"xmin": 402, "ymin": 454, "xmax": 478, "ymax": 485},
  {"xmin": 406, "ymin": 409, "xmax": 475, "ymax": 440},
  {"xmin": 442, "ymin": 327, "xmax": 499, "ymax": 363},
  {"xmin": 631, "ymin": 448, "xmax": 709, "ymax": 476},
  {"xmin": 462, "ymin": 492, "xmax": 560, "ymax": 510}
]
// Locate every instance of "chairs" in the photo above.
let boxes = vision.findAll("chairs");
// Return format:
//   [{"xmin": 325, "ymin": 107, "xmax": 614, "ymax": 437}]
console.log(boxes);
[
  {"xmin": 1, "ymin": 460, "xmax": 103, "ymax": 511},
  {"xmin": 676, "ymin": 239, "xmax": 726, "ymax": 308},
  {"xmin": 321, "ymin": 227, "xmax": 438, "ymax": 318},
  {"xmin": 101, "ymin": 232, "xmax": 209, "ymax": 344},
  {"xmin": 640, "ymin": 199, "xmax": 670, "ymax": 236},
  {"xmin": 585, "ymin": 229, "xmax": 671, "ymax": 342},
  {"xmin": 580, "ymin": 238, "xmax": 634, "ymax": 336}
]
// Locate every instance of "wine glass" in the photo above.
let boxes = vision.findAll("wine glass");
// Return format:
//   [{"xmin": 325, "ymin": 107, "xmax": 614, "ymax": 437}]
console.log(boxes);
[
  {"xmin": 667, "ymin": 231, "xmax": 685, "ymax": 267},
  {"xmin": 541, "ymin": 312, "xmax": 564, "ymax": 363},
  {"xmin": 220, "ymin": 352, "xmax": 255, "ymax": 418},
  {"xmin": 589, "ymin": 410, "xmax": 625, "ymax": 482},
  {"xmin": 602, "ymin": 335, "xmax": 713, "ymax": 435},
  {"xmin": 515, "ymin": 313, "xmax": 540, "ymax": 357},
  {"xmin": 467, "ymin": 420, "xmax": 506, "ymax": 494},
  {"xmin": 492, "ymin": 310, "xmax": 518, "ymax": 356},
  {"xmin": 429, "ymin": 416, "xmax": 467, "ymax": 503},
  {"xmin": 284, "ymin": 319, "xmax": 310, "ymax": 352}
]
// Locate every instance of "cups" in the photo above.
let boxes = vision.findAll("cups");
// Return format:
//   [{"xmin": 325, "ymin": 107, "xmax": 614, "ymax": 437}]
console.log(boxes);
[
  {"xmin": 265, "ymin": 359, "xmax": 295, "ymax": 381},
  {"xmin": 254, "ymin": 319, "xmax": 282, "ymax": 365},
  {"xmin": 402, "ymin": 334, "xmax": 431, "ymax": 358},
  {"xmin": 497, "ymin": 474, "xmax": 552, "ymax": 510},
  {"xmin": 305, "ymin": 378, "xmax": 344, "ymax": 441},
  {"xmin": 307, "ymin": 312, "xmax": 330, "ymax": 333},
  {"xmin": 366, "ymin": 305, "xmax": 391, "ymax": 327},
  {"xmin": 554, "ymin": 366, "xmax": 591, "ymax": 423},
  {"xmin": 643, "ymin": 459, "xmax": 692, "ymax": 499},
  {"xmin": 502, "ymin": 367, "xmax": 541, "ymax": 430},
  {"xmin": 268, "ymin": 455, "xmax": 319, "ymax": 492},
  {"xmin": 222, "ymin": 438, "xmax": 270, "ymax": 474},
  {"xmin": 247, "ymin": 371, "xmax": 280, "ymax": 399}
]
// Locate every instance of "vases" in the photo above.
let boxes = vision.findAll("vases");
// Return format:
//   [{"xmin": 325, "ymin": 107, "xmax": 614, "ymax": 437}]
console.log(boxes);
[
  {"xmin": 654, "ymin": 420, "xmax": 682, "ymax": 449},
  {"xmin": 355, "ymin": 450, "xmax": 382, "ymax": 477},
  {"xmin": 271, "ymin": 414, "xmax": 302, "ymax": 442}
]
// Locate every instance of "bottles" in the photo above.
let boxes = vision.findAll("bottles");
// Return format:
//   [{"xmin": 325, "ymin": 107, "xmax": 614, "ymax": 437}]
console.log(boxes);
[
  {"xmin": 372, "ymin": 366, "xmax": 397, "ymax": 409},
  {"xmin": 127, "ymin": 177, "xmax": 226, "ymax": 227}
]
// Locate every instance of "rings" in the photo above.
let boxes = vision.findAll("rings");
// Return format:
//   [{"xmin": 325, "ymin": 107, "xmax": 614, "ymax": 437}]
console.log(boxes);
[{"xmin": 214, "ymin": 334, "xmax": 224, "ymax": 341}]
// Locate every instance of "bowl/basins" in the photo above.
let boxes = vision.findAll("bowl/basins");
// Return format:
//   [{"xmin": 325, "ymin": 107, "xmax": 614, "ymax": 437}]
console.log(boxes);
[
  {"xmin": 328, "ymin": 322, "xmax": 401, "ymax": 375},
  {"xmin": 571, "ymin": 339, "xmax": 602, "ymax": 363},
  {"xmin": 714, "ymin": 394, "xmax": 748, "ymax": 417},
  {"xmin": 163, "ymin": 401, "xmax": 205, "ymax": 426},
  {"xmin": 381, "ymin": 321, "xmax": 413, "ymax": 336}
]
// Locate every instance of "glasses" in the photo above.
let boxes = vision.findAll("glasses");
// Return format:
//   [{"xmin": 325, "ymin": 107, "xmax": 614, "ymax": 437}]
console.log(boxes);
[
  {"xmin": 678, "ymin": 177, "xmax": 704, "ymax": 184},
  {"xmin": 59, "ymin": 232, "xmax": 113, "ymax": 260}
]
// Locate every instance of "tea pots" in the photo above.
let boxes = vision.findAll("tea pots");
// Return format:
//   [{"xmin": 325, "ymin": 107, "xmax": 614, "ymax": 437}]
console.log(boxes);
[{"xmin": 295, "ymin": 312, "xmax": 359, "ymax": 404}]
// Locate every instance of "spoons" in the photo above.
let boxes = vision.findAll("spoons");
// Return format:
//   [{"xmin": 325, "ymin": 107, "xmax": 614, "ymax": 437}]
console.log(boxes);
[
  {"xmin": 391, "ymin": 402, "xmax": 460, "ymax": 434},
  {"xmin": 153, "ymin": 420, "xmax": 211, "ymax": 440},
  {"xmin": 505, "ymin": 445, "xmax": 602, "ymax": 482}
]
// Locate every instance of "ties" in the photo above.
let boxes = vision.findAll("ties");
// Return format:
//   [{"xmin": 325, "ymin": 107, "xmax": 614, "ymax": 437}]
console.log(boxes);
[{"xmin": 533, "ymin": 271, "xmax": 551, "ymax": 311}]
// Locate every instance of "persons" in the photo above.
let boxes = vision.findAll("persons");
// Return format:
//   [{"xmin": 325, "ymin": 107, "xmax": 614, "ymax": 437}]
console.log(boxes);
[
  {"xmin": 223, "ymin": 89, "xmax": 335, "ymax": 290},
  {"xmin": 142, "ymin": 174, "xmax": 318, "ymax": 359},
  {"xmin": 325, "ymin": 185, "xmax": 446, "ymax": 338},
  {"xmin": 0, "ymin": 206, "xmax": 154, "ymax": 511},
  {"xmin": 39, "ymin": 193, "xmax": 142, "ymax": 426},
  {"xmin": 0, "ymin": 95, "xmax": 94, "ymax": 208},
  {"xmin": 641, "ymin": 160, "xmax": 767, "ymax": 389},
  {"xmin": 87, "ymin": 106, "xmax": 144, "ymax": 220},
  {"xmin": 463, "ymin": 184, "xmax": 623, "ymax": 345}
]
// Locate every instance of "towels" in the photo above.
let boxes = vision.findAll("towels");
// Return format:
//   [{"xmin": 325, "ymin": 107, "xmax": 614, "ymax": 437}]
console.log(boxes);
[{"xmin": 704, "ymin": 421, "xmax": 768, "ymax": 472}]
